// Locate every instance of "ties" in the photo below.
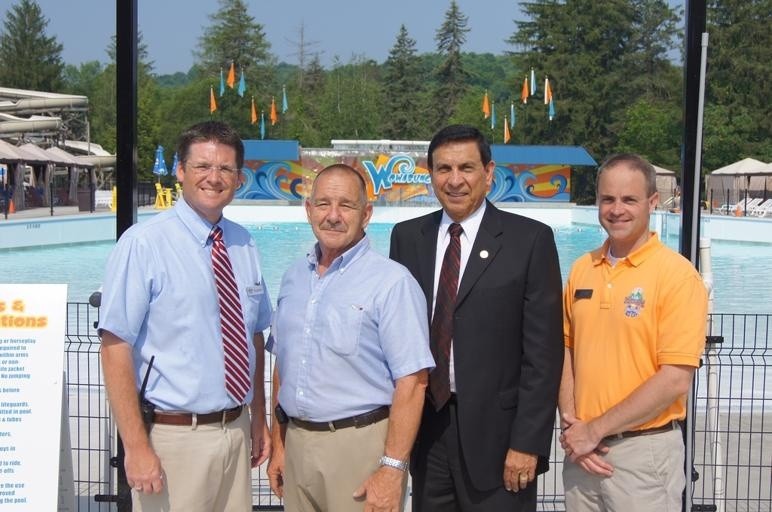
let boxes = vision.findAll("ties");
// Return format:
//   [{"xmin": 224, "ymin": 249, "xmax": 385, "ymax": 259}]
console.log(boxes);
[
  {"xmin": 207, "ymin": 224, "xmax": 252, "ymax": 407},
  {"xmin": 424, "ymin": 223, "xmax": 467, "ymax": 416}
]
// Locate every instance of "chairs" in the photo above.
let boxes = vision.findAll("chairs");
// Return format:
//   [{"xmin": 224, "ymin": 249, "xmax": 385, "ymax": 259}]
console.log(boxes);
[{"xmin": 719, "ymin": 197, "xmax": 772, "ymax": 217}]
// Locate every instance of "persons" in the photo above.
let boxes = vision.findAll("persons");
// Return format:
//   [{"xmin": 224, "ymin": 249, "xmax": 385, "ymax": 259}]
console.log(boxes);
[
  {"xmin": 388, "ymin": 122, "xmax": 565, "ymax": 511},
  {"xmin": 264, "ymin": 163, "xmax": 437, "ymax": 511},
  {"xmin": 555, "ymin": 152, "xmax": 710, "ymax": 511},
  {"xmin": 95, "ymin": 117, "xmax": 274, "ymax": 512}
]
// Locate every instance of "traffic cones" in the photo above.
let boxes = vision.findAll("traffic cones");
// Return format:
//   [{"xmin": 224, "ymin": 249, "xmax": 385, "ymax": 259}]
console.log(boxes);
[
  {"xmin": 9, "ymin": 199, "xmax": 15, "ymax": 214},
  {"xmin": 735, "ymin": 205, "xmax": 742, "ymax": 217}
]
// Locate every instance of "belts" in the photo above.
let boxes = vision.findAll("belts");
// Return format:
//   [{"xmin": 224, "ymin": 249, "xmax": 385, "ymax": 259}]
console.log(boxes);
[
  {"xmin": 287, "ymin": 405, "xmax": 390, "ymax": 432},
  {"xmin": 151, "ymin": 404, "xmax": 247, "ymax": 428},
  {"xmin": 601, "ymin": 418, "xmax": 673, "ymax": 440}
]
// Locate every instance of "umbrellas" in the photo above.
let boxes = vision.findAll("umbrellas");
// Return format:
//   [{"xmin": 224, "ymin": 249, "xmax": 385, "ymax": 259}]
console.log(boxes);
[
  {"xmin": 152, "ymin": 144, "xmax": 169, "ymax": 183},
  {"xmin": 171, "ymin": 152, "xmax": 178, "ymax": 183}
]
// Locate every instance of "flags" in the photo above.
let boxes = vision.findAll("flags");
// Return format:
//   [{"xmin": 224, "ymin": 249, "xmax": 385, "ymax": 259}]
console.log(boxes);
[
  {"xmin": 210, "ymin": 62, "xmax": 288, "ymax": 140},
  {"xmin": 482, "ymin": 68, "xmax": 556, "ymax": 143}
]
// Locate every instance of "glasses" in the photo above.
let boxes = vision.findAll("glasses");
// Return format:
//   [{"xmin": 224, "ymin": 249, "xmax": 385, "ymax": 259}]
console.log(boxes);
[{"xmin": 185, "ymin": 160, "xmax": 241, "ymax": 175}]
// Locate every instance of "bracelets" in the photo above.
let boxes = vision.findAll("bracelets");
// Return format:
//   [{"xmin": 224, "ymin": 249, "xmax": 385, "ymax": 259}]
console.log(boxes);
[
  {"xmin": 561, "ymin": 425, "xmax": 571, "ymax": 434},
  {"xmin": 377, "ymin": 454, "xmax": 410, "ymax": 473}
]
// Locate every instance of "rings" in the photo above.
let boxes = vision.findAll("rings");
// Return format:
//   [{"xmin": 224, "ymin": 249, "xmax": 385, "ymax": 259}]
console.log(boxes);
[
  {"xmin": 135, "ymin": 485, "xmax": 142, "ymax": 490},
  {"xmin": 519, "ymin": 474, "xmax": 529, "ymax": 481}
]
